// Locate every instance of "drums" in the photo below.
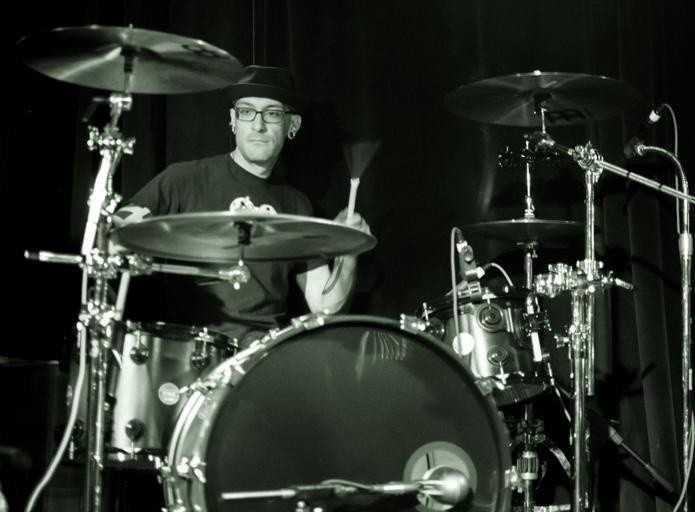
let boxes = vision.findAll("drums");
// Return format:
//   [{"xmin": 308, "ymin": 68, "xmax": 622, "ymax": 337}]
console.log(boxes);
[
  {"xmin": 420, "ymin": 285, "xmax": 547, "ymax": 406},
  {"xmin": 160, "ymin": 313, "xmax": 512, "ymax": 511},
  {"xmin": 65, "ymin": 319, "xmax": 241, "ymax": 472}
]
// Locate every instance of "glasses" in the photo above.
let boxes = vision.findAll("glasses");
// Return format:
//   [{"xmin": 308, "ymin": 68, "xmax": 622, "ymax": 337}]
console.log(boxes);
[{"xmin": 235, "ymin": 106, "xmax": 296, "ymax": 123}]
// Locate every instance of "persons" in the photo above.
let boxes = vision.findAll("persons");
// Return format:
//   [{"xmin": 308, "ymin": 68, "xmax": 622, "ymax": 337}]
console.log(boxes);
[{"xmin": 114, "ymin": 62, "xmax": 372, "ymax": 359}]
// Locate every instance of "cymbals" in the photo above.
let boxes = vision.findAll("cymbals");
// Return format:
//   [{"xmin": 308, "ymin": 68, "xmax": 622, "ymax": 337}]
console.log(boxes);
[
  {"xmin": 14, "ymin": 24, "xmax": 244, "ymax": 94},
  {"xmin": 111, "ymin": 210, "xmax": 378, "ymax": 261},
  {"xmin": 466, "ymin": 219, "xmax": 581, "ymax": 238},
  {"xmin": 443, "ymin": 72, "xmax": 641, "ymax": 128}
]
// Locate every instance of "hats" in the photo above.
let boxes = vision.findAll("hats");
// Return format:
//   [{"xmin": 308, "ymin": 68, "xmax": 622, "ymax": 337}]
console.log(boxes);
[{"xmin": 222, "ymin": 64, "xmax": 303, "ymax": 110}]
